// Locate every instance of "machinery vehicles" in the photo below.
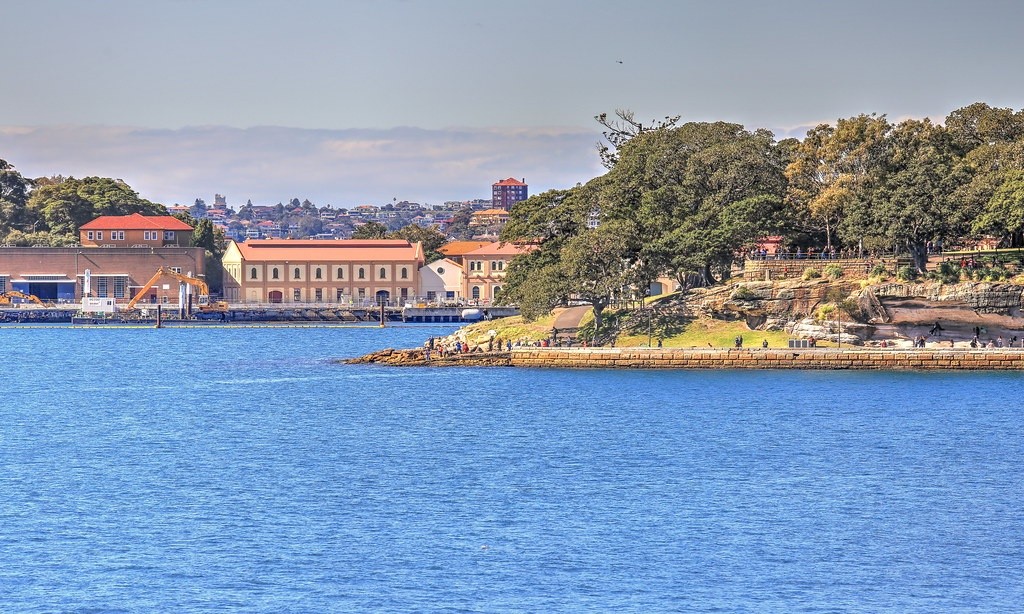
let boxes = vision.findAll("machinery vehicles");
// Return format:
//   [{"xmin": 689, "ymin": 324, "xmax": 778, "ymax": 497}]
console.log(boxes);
[
  {"xmin": 0, "ymin": 290, "xmax": 56, "ymax": 308},
  {"xmin": 122, "ymin": 265, "xmax": 229, "ymax": 313}
]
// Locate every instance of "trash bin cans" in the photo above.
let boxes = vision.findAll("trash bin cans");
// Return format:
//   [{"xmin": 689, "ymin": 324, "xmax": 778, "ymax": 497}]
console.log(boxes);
[{"xmin": 788, "ymin": 339, "xmax": 809, "ymax": 348}]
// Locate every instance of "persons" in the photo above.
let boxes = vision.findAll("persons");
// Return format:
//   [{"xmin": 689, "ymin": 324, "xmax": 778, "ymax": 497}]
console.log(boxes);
[
  {"xmin": 913, "ymin": 335, "xmax": 926, "ymax": 348},
  {"xmin": 611, "ymin": 339, "xmax": 615, "ymax": 347},
  {"xmin": 735, "ymin": 335, "xmax": 743, "ymax": 347},
  {"xmin": 1008, "ymin": 338, "xmax": 1013, "ymax": 347},
  {"xmin": 566, "ymin": 336, "xmax": 574, "ymax": 347},
  {"xmin": 581, "ymin": 338, "xmax": 607, "ymax": 347},
  {"xmin": 948, "ymin": 339, "xmax": 954, "ymax": 347},
  {"xmin": 933, "ymin": 322, "xmax": 942, "ymax": 336},
  {"xmin": 524, "ymin": 337, "xmax": 528, "ymax": 347},
  {"xmin": 809, "ymin": 336, "xmax": 817, "ymax": 347},
  {"xmin": 734, "ymin": 244, "xmax": 867, "ymax": 259},
  {"xmin": 423, "ymin": 334, "xmax": 520, "ymax": 361},
  {"xmin": 551, "ymin": 326, "xmax": 559, "ymax": 342},
  {"xmin": 762, "ymin": 339, "xmax": 768, "ymax": 348},
  {"xmin": 533, "ymin": 336, "xmax": 562, "ymax": 347},
  {"xmin": 970, "ymin": 339, "xmax": 978, "ymax": 348},
  {"xmin": 975, "ymin": 326, "xmax": 980, "ymax": 339},
  {"xmin": 882, "ymin": 339, "xmax": 887, "ymax": 348},
  {"xmin": 988, "ymin": 340, "xmax": 994, "ymax": 348},
  {"xmin": 998, "ymin": 336, "xmax": 1002, "ymax": 347},
  {"xmin": 657, "ymin": 340, "xmax": 662, "ymax": 347},
  {"xmin": 945, "ymin": 257, "xmax": 978, "ymax": 269}
]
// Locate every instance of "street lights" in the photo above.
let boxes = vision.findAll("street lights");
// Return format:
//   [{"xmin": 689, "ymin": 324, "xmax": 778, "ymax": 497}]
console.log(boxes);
[
  {"xmin": 836, "ymin": 299, "xmax": 844, "ymax": 348},
  {"xmin": 646, "ymin": 308, "xmax": 653, "ymax": 347}
]
